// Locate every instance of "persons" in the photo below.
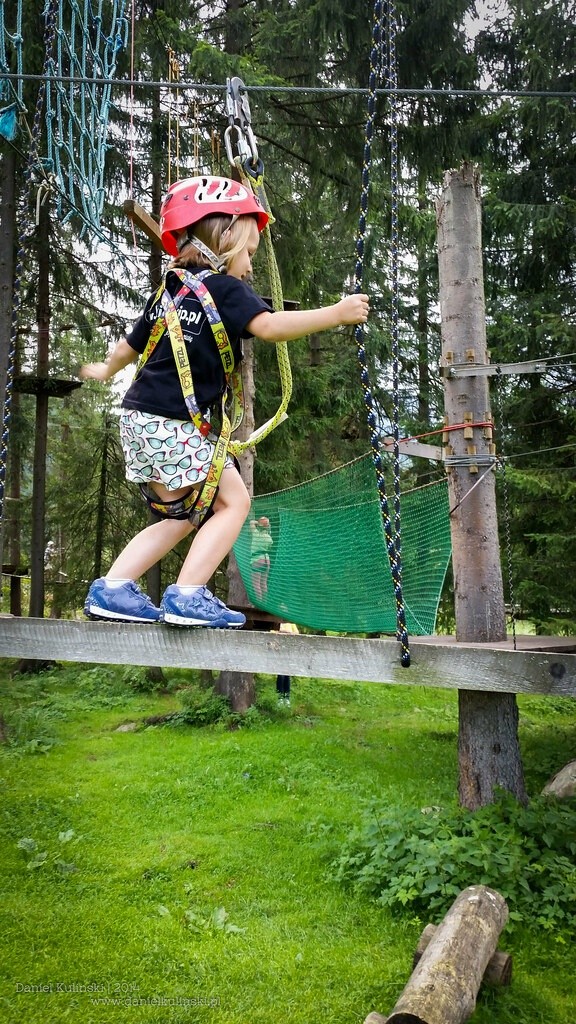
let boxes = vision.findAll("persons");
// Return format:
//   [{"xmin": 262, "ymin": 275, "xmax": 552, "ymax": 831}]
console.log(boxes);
[
  {"xmin": 270, "ymin": 605, "xmax": 299, "ymax": 707},
  {"xmin": 44, "ymin": 541, "xmax": 58, "ymax": 567},
  {"xmin": 250, "ymin": 517, "xmax": 273, "ymax": 604},
  {"xmin": 81, "ymin": 175, "xmax": 369, "ymax": 628}
]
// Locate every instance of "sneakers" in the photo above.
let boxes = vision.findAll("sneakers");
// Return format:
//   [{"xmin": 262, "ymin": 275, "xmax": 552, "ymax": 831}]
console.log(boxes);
[
  {"xmin": 159, "ymin": 583, "xmax": 246, "ymax": 626},
  {"xmin": 83, "ymin": 579, "xmax": 160, "ymax": 623}
]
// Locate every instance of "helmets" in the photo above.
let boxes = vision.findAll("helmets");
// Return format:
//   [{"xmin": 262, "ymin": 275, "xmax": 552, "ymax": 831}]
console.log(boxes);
[
  {"xmin": 258, "ymin": 517, "xmax": 270, "ymax": 528},
  {"xmin": 160, "ymin": 176, "xmax": 270, "ymax": 258}
]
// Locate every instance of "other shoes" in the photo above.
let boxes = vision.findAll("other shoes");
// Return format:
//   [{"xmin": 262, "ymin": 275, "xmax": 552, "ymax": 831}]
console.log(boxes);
[{"xmin": 276, "ymin": 699, "xmax": 291, "ymax": 708}]
